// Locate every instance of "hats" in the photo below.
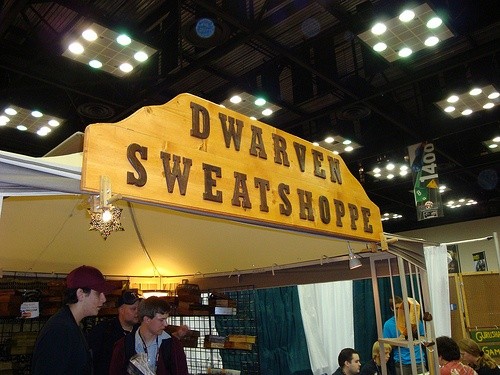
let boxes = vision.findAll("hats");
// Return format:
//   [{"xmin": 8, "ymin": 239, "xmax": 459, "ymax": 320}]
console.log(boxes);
[
  {"xmin": 116, "ymin": 291, "xmax": 143, "ymax": 306},
  {"xmin": 65, "ymin": 265, "xmax": 114, "ymax": 293}
]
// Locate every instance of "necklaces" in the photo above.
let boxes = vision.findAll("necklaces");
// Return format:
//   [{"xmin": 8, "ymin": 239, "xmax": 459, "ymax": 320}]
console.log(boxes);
[
  {"xmin": 375, "ymin": 362, "xmax": 383, "ymax": 375},
  {"xmin": 138, "ymin": 328, "xmax": 158, "ymax": 366}
]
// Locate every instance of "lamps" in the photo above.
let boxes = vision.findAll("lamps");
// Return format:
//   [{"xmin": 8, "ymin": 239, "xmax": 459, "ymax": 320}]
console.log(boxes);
[
  {"xmin": 483, "ymin": 136, "xmax": 500, "ymax": 153},
  {"xmin": 381, "ymin": 212, "xmax": 404, "ymax": 222},
  {"xmin": 0, "ymin": 101, "xmax": 67, "ymax": 140},
  {"xmin": 443, "ymin": 197, "xmax": 478, "ymax": 209},
  {"xmin": 218, "ymin": 91, "xmax": 283, "ymax": 121},
  {"xmin": 317, "ymin": 135, "xmax": 364, "ymax": 154},
  {"xmin": 87, "ymin": 194, "xmax": 126, "ymax": 241},
  {"xmin": 366, "ymin": 162, "xmax": 412, "ymax": 181},
  {"xmin": 60, "ymin": 23, "xmax": 157, "ymax": 82},
  {"xmin": 434, "ymin": 84, "xmax": 500, "ymax": 120},
  {"xmin": 411, "ymin": 182, "xmax": 453, "ymax": 199},
  {"xmin": 345, "ymin": 241, "xmax": 362, "ymax": 269}
]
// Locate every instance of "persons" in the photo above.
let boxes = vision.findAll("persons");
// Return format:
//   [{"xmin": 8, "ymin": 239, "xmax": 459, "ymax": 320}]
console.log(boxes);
[
  {"xmin": 332, "ymin": 348, "xmax": 361, "ymax": 375},
  {"xmin": 30, "ymin": 265, "xmax": 123, "ymax": 375},
  {"xmin": 359, "ymin": 341, "xmax": 392, "ymax": 375},
  {"xmin": 459, "ymin": 337, "xmax": 500, "ymax": 375},
  {"xmin": 436, "ymin": 336, "xmax": 478, "ymax": 375},
  {"xmin": 383, "ymin": 296, "xmax": 426, "ymax": 375},
  {"xmin": 87, "ymin": 291, "xmax": 189, "ymax": 375},
  {"xmin": 110, "ymin": 296, "xmax": 188, "ymax": 375}
]
